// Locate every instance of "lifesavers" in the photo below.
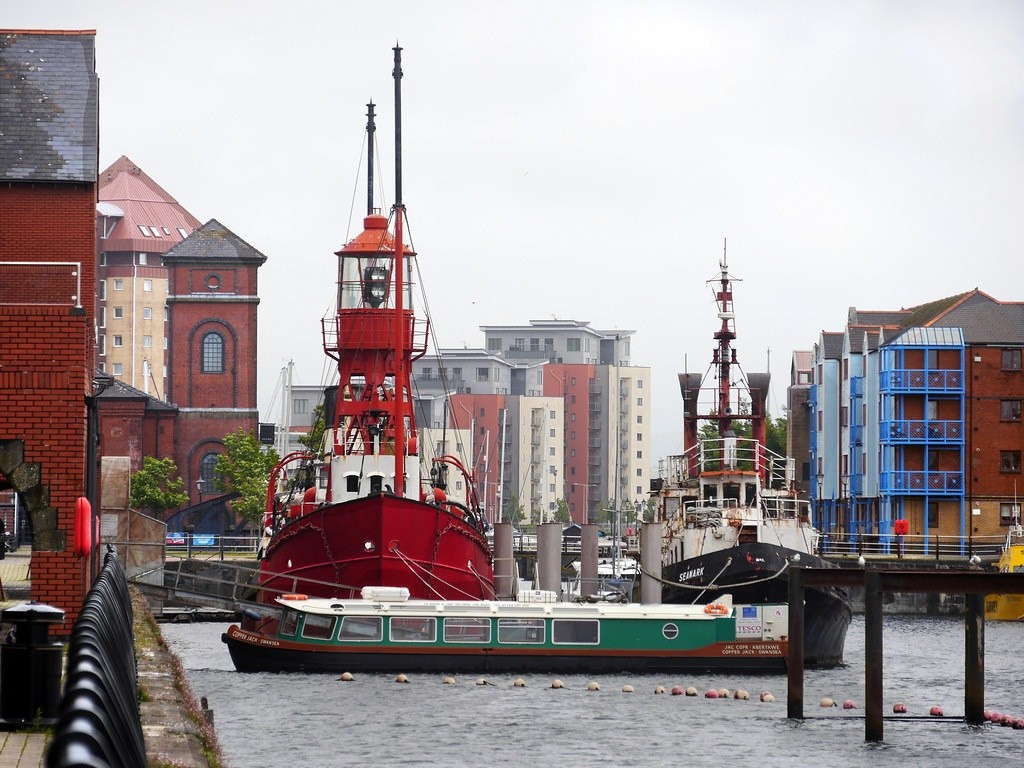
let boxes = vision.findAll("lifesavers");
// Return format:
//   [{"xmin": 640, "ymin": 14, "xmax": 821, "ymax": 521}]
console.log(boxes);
[
  {"xmin": 282, "ymin": 593, "xmax": 308, "ymax": 600},
  {"xmin": 704, "ymin": 604, "xmax": 728, "ymax": 614},
  {"xmin": 264, "ymin": 514, "xmax": 285, "ymax": 537}
]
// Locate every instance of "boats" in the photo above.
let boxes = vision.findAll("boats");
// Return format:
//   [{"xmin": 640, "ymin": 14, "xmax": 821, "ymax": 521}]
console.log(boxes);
[
  {"xmin": 220, "ymin": 584, "xmax": 808, "ymax": 677},
  {"xmin": 571, "ymin": 236, "xmax": 856, "ymax": 672},
  {"xmin": 985, "ymin": 477, "xmax": 1024, "ymax": 624},
  {"xmin": 252, "ymin": 37, "xmax": 499, "ymax": 644}
]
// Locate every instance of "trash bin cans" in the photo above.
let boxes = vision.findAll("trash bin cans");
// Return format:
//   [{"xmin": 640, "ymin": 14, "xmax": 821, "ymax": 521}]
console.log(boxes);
[{"xmin": 0, "ymin": 598, "xmax": 69, "ymax": 730}]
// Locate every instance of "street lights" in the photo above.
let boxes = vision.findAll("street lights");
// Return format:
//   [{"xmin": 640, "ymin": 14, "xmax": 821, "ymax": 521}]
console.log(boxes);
[
  {"xmin": 634, "ymin": 498, "xmax": 639, "ymax": 535},
  {"xmin": 607, "ymin": 496, "xmax": 616, "ymax": 539},
  {"xmin": 195, "ymin": 475, "xmax": 207, "ymax": 533},
  {"xmin": 624, "ymin": 497, "xmax": 631, "ymax": 534},
  {"xmin": 640, "ymin": 496, "xmax": 646, "ymax": 519}
]
